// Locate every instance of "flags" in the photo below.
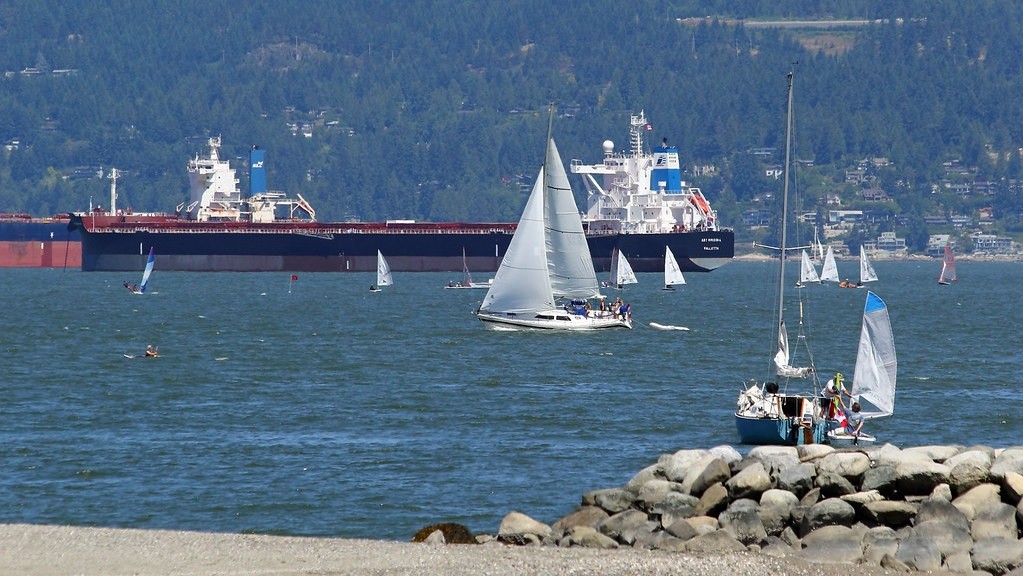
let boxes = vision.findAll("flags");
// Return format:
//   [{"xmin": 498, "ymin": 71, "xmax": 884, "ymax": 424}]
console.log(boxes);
[
  {"xmin": 644, "ymin": 124, "xmax": 652, "ymax": 131},
  {"xmin": 291, "ymin": 276, "xmax": 297, "ymax": 280}
]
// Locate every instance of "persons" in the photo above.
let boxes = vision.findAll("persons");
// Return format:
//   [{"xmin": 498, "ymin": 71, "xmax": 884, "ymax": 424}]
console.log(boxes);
[
  {"xmin": 448, "ymin": 281, "xmax": 463, "ymax": 287},
  {"xmin": 819, "ymin": 375, "xmax": 864, "ymax": 436},
  {"xmin": 671, "ymin": 223, "xmax": 706, "ymax": 233},
  {"xmin": 146, "ymin": 345, "xmax": 156, "ymax": 358},
  {"xmin": 661, "ymin": 137, "xmax": 668, "ymax": 149},
  {"xmin": 844, "ymin": 279, "xmax": 862, "ymax": 288},
  {"xmin": 584, "ymin": 280, "xmax": 632, "ymax": 324},
  {"xmin": 370, "ymin": 284, "xmax": 375, "ymax": 290},
  {"xmin": 123, "ymin": 281, "xmax": 140, "ymax": 292}
]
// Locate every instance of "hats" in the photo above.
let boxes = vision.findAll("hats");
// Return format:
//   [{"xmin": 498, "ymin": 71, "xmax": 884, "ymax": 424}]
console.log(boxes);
[{"xmin": 834, "ymin": 373, "xmax": 845, "ymax": 381}]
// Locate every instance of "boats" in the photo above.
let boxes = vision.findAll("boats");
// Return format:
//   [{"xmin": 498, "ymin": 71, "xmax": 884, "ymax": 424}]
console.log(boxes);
[
  {"xmin": 0, "ymin": 210, "xmax": 86, "ymax": 268},
  {"xmin": 66, "ymin": 106, "xmax": 738, "ymax": 274},
  {"xmin": 443, "ymin": 244, "xmax": 496, "ymax": 288}
]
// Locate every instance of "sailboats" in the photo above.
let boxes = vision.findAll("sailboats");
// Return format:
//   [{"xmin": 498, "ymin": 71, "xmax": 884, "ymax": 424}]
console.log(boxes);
[
  {"xmin": 856, "ymin": 245, "xmax": 880, "ymax": 289},
  {"xmin": 938, "ymin": 246, "xmax": 958, "ymax": 286},
  {"xmin": 827, "ymin": 290, "xmax": 897, "ymax": 445},
  {"xmin": 815, "ymin": 245, "xmax": 840, "ymax": 286},
  {"xmin": 734, "ymin": 64, "xmax": 842, "ymax": 447},
  {"xmin": 601, "ymin": 247, "xmax": 639, "ymax": 291},
  {"xmin": 367, "ymin": 249, "xmax": 393, "ymax": 293},
  {"xmin": 467, "ymin": 134, "xmax": 633, "ymax": 332},
  {"xmin": 662, "ymin": 245, "xmax": 687, "ymax": 290},
  {"xmin": 124, "ymin": 246, "xmax": 155, "ymax": 296},
  {"xmin": 794, "ymin": 249, "xmax": 822, "ymax": 290}
]
